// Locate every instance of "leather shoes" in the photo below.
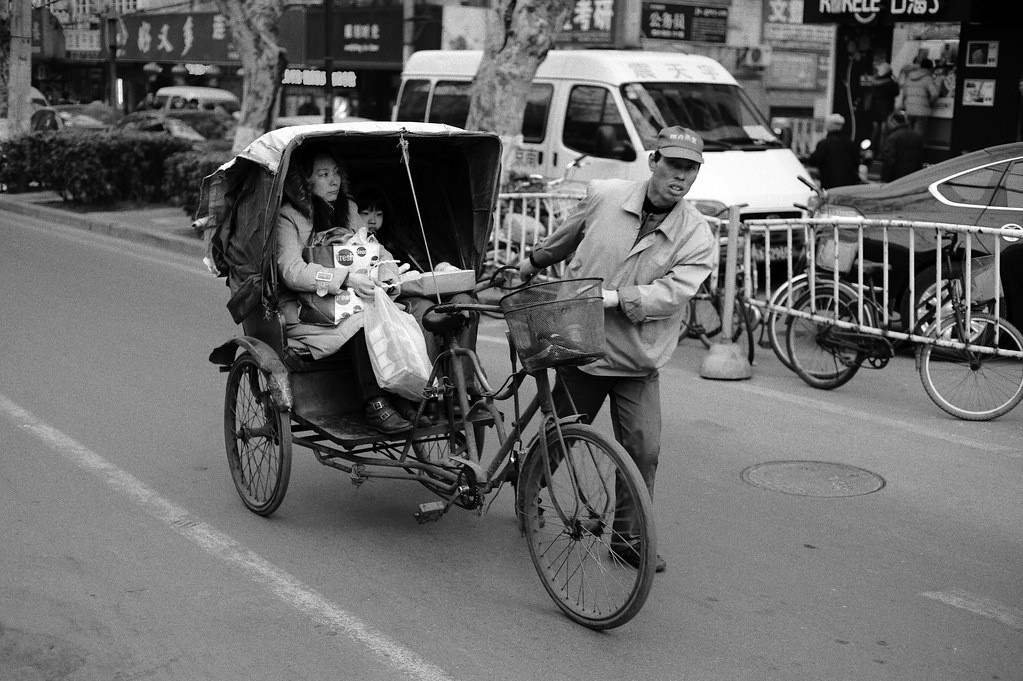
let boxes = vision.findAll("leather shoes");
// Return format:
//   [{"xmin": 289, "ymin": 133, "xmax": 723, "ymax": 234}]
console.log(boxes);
[
  {"xmin": 362, "ymin": 402, "xmax": 413, "ymax": 434},
  {"xmin": 394, "ymin": 406, "xmax": 431, "ymax": 427}
]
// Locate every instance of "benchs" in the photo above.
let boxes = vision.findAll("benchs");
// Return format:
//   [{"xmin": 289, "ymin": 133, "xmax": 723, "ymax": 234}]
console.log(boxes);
[{"xmin": 283, "ymin": 244, "xmax": 473, "ymax": 373}]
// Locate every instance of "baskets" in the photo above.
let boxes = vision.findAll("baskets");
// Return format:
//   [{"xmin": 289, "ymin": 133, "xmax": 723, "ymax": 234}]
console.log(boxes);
[
  {"xmin": 962, "ymin": 255, "xmax": 1004, "ymax": 304},
  {"xmin": 816, "ymin": 226, "xmax": 858, "ymax": 274},
  {"xmin": 498, "ymin": 278, "xmax": 608, "ymax": 373}
]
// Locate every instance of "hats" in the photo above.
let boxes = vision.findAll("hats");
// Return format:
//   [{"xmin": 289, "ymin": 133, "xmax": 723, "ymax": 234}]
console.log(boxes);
[
  {"xmin": 876, "ymin": 62, "xmax": 889, "ymax": 76},
  {"xmin": 654, "ymin": 126, "xmax": 704, "ymax": 164}
]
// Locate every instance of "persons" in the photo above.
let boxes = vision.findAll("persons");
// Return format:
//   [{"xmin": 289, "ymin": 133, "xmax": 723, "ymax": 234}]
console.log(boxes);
[
  {"xmin": 276, "ymin": 148, "xmax": 494, "ymax": 435},
  {"xmin": 840, "ymin": 34, "xmax": 900, "ymax": 161},
  {"xmin": 297, "ymin": 94, "xmax": 322, "ymax": 116},
  {"xmin": 510, "ymin": 125, "xmax": 714, "ymax": 573},
  {"xmin": 798, "ymin": 112, "xmax": 861, "ymax": 190},
  {"xmin": 897, "ymin": 58, "xmax": 939, "ymax": 134},
  {"xmin": 878, "ymin": 111, "xmax": 924, "ymax": 184}
]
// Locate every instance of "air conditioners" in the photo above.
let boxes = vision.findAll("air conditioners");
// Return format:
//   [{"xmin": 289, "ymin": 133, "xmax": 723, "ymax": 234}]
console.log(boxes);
[{"xmin": 743, "ymin": 44, "xmax": 772, "ymax": 67}]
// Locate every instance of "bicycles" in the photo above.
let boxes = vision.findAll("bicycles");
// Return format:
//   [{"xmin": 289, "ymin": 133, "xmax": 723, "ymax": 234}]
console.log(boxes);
[
  {"xmin": 478, "ymin": 153, "xmax": 877, "ymax": 379},
  {"xmin": 784, "ymin": 229, "xmax": 1022, "ymax": 419}
]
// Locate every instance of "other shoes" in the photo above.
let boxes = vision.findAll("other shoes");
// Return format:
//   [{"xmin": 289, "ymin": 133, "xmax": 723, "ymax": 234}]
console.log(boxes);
[
  {"xmin": 423, "ymin": 396, "xmax": 439, "ymax": 424},
  {"xmin": 442, "ymin": 399, "xmax": 461, "ymax": 418},
  {"xmin": 538, "ymin": 505, "xmax": 545, "ymax": 528},
  {"xmin": 608, "ymin": 544, "xmax": 666, "ymax": 572}
]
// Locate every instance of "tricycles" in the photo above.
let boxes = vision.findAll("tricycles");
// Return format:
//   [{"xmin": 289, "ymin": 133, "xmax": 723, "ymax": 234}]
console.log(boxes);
[{"xmin": 196, "ymin": 116, "xmax": 657, "ymax": 630}]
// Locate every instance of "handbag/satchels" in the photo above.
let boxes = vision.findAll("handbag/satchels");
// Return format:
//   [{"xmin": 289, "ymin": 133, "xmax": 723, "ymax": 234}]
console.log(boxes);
[
  {"xmin": 397, "ymin": 270, "xmax": 476, "ymax": 296},
  {"xmin": 362, "ymin": 285, "xmax": 439, "ymax": 402},
  {"xmin": 297, "ymin": 226, "xmax": 380, "ymax": 326}
]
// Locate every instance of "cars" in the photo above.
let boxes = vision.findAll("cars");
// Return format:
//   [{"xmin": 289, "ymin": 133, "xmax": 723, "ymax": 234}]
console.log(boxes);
[
  {"xmin": 805, "ymin": 141, "xmax": 1023, "ymax": 362},
  {"xmin": 30, "ymin": 105, "xmax": 127, "ymax": 132},
  {"xmin": 109, "ymin": 110, "xmax": 238, "ymax": 142},
  {"xmin": 277, "ymin": 114, "xmax": 378, "ymax": 128}
]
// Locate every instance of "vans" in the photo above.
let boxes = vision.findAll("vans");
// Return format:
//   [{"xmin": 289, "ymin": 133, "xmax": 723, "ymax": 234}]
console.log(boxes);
[
  {"xmin": 31, "ymin": 85, "xmax": 49, "ymax": 111},
  {"xmin": 147, "ymin": 87, "xmax": 240, "ymax": 115},
  {"xmin": 392, "ymin": 48, "xmax": 821, "ymax": 278}
]
